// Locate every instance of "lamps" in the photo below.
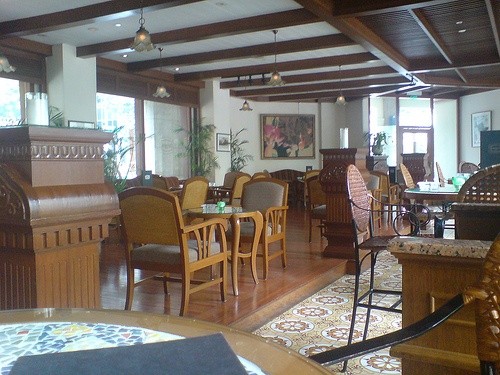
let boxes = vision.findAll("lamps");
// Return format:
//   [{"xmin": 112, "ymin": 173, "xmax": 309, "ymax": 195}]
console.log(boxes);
[
  {"xmin": 153, "ymin": 47, "xmax": 170, "ymax": 97},
  {"xmin": 240, "ymin": 75, "xmax": 252, "ymax": 111},
  {"xmin": 129, "ymin": 0, "xmax": 156, "ymax": 50},
  {"xmin": 268, "ymin": 30, "xmax": 287, "ymax": 85},
  {"xmin": 0, "ymin": 56, "xmax": 16, "ymax": 73},
  {"xmin": 336, "ymin": 63, "xmax": 348, "ymax": 105}
]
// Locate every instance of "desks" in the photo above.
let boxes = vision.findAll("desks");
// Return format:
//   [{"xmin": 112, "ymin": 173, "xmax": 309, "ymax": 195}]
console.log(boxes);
[
  {"xmin": 403, "ymin": 182, "xmax": 500, "ymax": 238},
  {"xmin": 183, "ymin": 204, "xmax": 246, "ymax": 280},
  {"xmin": 207, "ymin": 187, "xmax": 231, "ymax": 203},
  {"xmin": 0, "ymin": 303, "xmax": 335, "ymax": 374}
]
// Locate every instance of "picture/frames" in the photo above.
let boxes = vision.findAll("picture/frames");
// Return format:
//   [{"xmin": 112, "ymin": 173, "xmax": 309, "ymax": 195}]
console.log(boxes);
[
  {"xmin": 216, "ymin": 133, "xmax": 231, "ymax": 152},
  {"xmin": 261, "ymin": 114, "xmax": 315, "ymax": 160},
  {"xmin": 470, "ymin": 110, "xmax": 492, "ymax": 147},
  {"xmin": 68, "ymin": 121, "xmax": 95, "ymax": 128}
]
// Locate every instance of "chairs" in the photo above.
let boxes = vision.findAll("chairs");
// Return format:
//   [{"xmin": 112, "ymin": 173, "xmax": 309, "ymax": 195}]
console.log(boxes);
[{"xmin": 111, "ymin": 162, "xmax": 500, "ymax": 318}]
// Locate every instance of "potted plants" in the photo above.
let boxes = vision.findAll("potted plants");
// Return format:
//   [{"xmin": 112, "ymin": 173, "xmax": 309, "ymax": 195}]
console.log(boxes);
[{"xmin": 363, "ymin": 130, "xmax": 388, "ymax": 155}]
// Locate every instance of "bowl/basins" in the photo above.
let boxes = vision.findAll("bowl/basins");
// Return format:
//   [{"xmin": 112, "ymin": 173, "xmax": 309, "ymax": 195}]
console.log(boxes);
[
  {"xmin": 217, "ymin": 202, "xmax": 225, "ymax": 207},
  {"xmin": 451, "ymin": 173, "xmax": 471, "ymax": 191}
]
